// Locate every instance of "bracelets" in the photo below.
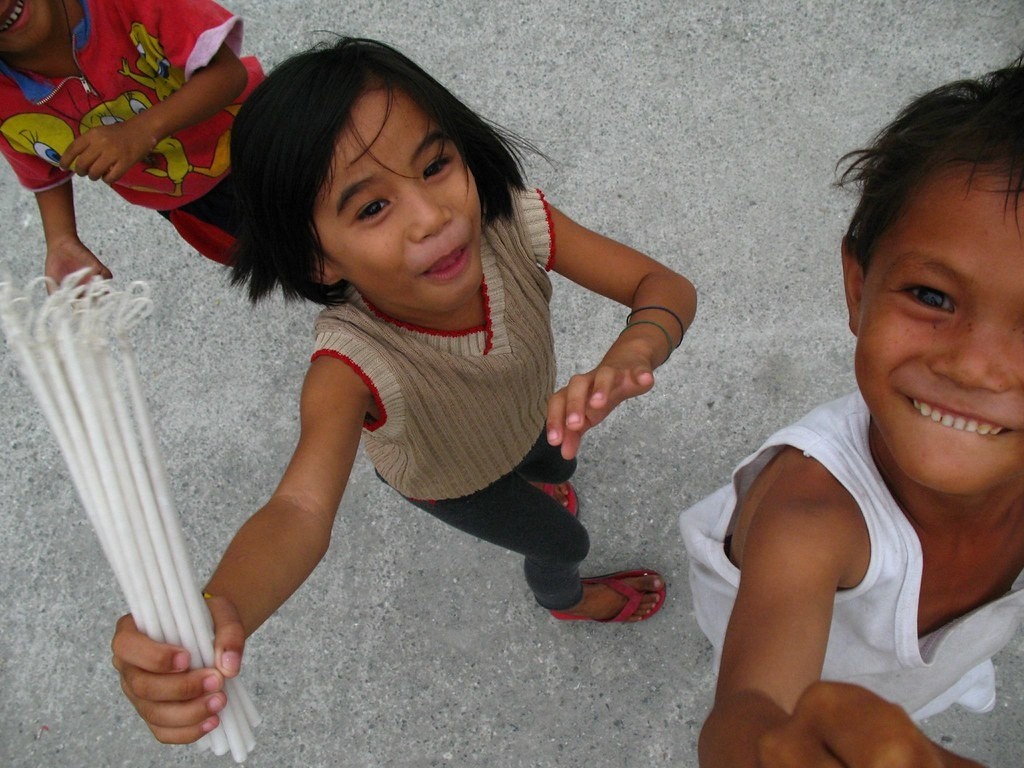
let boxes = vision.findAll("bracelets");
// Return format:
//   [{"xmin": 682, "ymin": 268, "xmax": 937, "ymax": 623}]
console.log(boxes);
[{"xmin": 619, "ymin": 307, "xmax": 684, "ymax": 365}]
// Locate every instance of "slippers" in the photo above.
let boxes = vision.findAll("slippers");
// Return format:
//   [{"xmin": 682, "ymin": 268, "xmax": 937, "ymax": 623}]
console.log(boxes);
[
  {"xmin": 530, "ymin": 480, "xmax": 578, "ymax": 515},
  {"xmin": 550, "ymin": 569, "xmax": 665, "ymax": 624}
]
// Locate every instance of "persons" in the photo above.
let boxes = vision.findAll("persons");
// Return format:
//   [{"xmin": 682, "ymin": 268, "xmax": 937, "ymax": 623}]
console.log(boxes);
[
  {"xmin": 111, "ymin": 32, "xmax": 698, "ymax": 744},
  {"xmin": 0, "ymin": 0, "xmax": 269, "ymax": 303},
  {"xmin": 680, "ymin": 47, "xmax": 1024, "ymax": 767}
]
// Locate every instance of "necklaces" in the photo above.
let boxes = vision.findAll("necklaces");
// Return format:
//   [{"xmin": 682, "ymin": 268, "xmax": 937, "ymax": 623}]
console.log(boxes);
[{"xmin": 35, "ymin": 0, "xmax": 158, "ymax": 169}]
